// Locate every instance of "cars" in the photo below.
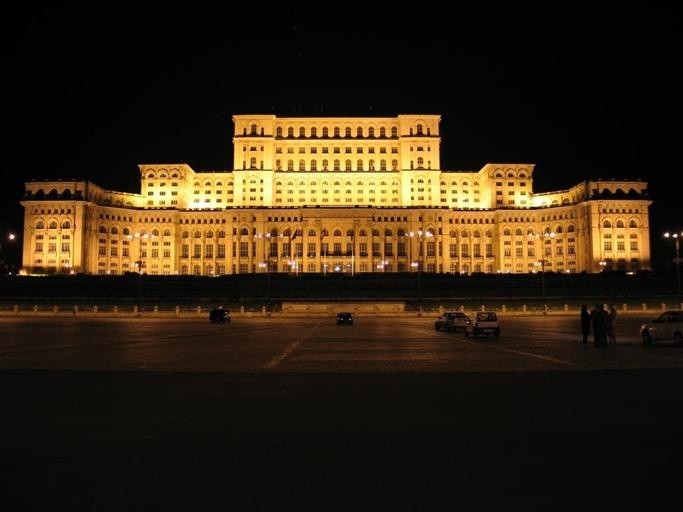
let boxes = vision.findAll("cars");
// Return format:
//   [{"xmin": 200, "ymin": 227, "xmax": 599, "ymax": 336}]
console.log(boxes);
[
  {"xmin": 336, "ymin": 311, "xmax": 353, "ymax": 325},
  {"xmin": 639, "ymin": 311, "xmax": 683, "ymax": 347},
  {"xmin": 210, "ymin": 308, "xmax": 231, "ymax": 324},
  {"xmin": 435, "ymin": 312, "xmax": 472, "ymax": 333}
]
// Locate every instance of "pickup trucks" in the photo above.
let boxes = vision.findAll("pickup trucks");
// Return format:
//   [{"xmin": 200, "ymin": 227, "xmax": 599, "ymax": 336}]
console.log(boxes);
[{"xmin": 471, "ymin": 311, "xmax": 500, "ymax": 338}]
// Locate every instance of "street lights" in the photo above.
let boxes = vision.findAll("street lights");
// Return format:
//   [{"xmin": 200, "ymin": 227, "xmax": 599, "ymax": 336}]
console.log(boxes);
[
  {"xmin": 663, "ymin": 231, "xmax": 683, "ymax": 294},
  {"xmin": 529, "ymin": 232, "xmax": 556, "ymax": 297},
  {"xmin": 128, "ymin": 232, "xmax": 155, "ymax": 297}
]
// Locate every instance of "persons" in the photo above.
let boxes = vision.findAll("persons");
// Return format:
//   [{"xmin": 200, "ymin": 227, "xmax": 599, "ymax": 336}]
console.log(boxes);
[{"xmin": 579, "ymin": 303, "xmax": 618, "ymax": 351}]
